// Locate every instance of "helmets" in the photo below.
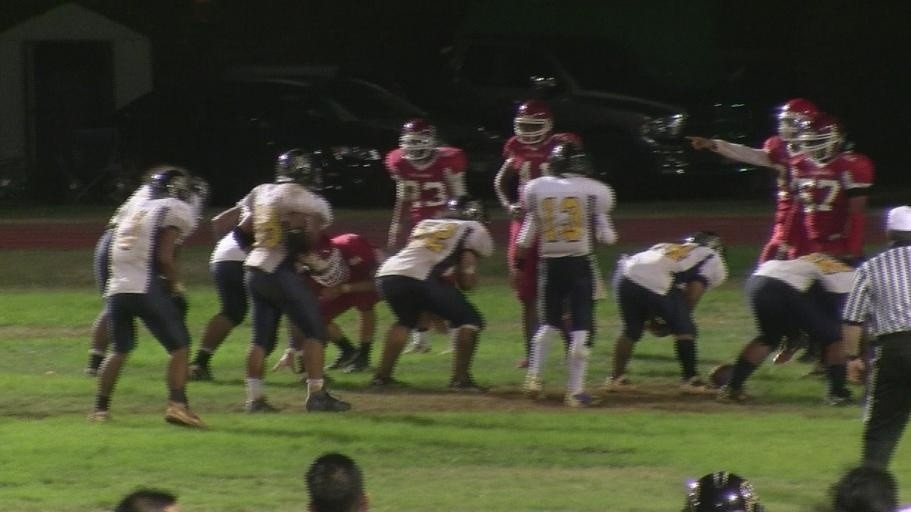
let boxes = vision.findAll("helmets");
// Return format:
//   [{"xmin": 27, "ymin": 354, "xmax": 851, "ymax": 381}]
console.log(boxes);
[
  {"xmin": 151, "ymin": 166, "xmax": 188, "ymax": 200},
  {"xmin": 778, "ymin": 99, "xmax": 818, "ymax": 142},
  {"xmin": 515, "ymin": 102, "xmax": 553, "ymax": 144},
  {"xmin": 803, "ymin": 117, "xmax": 839, "ymax": 160},
  {"xmin": 687, "ymin": 229, "xmax": 726, "ymax": 254},
  {"xmin": 448, "ymin": 194, "xmax": 486, "ymax": 225},
  {"xmin": 276, "ymin": 148, "xmax": 314, "ymax": 184},
  {"xmin": 550, "ymin": 143, "xmax": 587, "ymax": 174},
  {"xmin": 191, "ymin": 179, "xmax": 209, "ymax": 213},
  {"xmin": 401, "ymin": 120, "xmax": 437, "ymax": 160}
]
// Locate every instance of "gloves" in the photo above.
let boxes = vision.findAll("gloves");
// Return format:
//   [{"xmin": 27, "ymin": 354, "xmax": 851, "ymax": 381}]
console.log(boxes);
[{"xmin": 508, "ymin": 202, "xmax": 526, "ymax": 219}]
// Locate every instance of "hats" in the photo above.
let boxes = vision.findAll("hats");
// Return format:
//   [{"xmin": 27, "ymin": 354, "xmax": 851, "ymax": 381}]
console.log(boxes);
[{"xmin": 887, "ymin": 205, "xmax": 911, "ymax": 232}]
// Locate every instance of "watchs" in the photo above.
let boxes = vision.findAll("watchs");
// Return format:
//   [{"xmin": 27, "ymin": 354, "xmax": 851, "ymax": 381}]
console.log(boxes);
[{"xmin": 844, "ymin": 352, "xmax": 862, "ymax": 361}]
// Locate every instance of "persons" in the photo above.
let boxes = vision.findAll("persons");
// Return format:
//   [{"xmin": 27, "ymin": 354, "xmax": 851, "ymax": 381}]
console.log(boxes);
[
  {"xmin": 840, "ymin": 205, "xmax": 911, "ymax": 465},
  {"xmin": 112, "ymin": 486, "xmax": 180, "ymax": 512},
  {"xmin": 190, "ymin": 195, "xmax": 251, "ymax": 381},
  {"xmin": 87, "ymin": 167, "xmax": 188, "ymax": 378},
  {"xmin": 383, "ymin": 119, "xmax": 471, "ymax": 356},
  {"xmin": 495, "ymin": 100, "xmax": 585, "ymax": 369},
  {"xmin": 601, "ymin": 232, "xmax": 724, "ymax": 395},
  {"xmin": 786, "ymin": 110, "xmax": 875, "ymax": 382},
  {"xmin": 235, "ymin": 146, "xmax": 354, "ymax": 413},
  {"xmin": 512, "ymin": 139, "xmax": 616, "ymax": 410},
  {"xmin": 84, "ymin": 174, "xmax": 207, "ymax": 429},
  {"xmin": 305, "ymin": 451, "xmax": 369, "ymax": 511},
  {"xmin": 270, "ymin": 227, "xmax": 383, "ymax": 375},
  {"xmin": 684, "ymin": 98, "xmax": 819, "ymax": 268},
  {"xmin": 828, "ymin": 466, "xmax": 901, "ymax": 512},
  {"xmin": 368, "ymin": 195, "xmax": 493, "ymax": 394},
  {"xmin": 718, "ymin": 252, "xmax": 874, "ymax": 404}
]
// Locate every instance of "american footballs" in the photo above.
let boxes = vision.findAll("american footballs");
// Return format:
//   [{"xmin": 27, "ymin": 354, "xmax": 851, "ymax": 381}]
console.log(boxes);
[{"xmin": 709, "ymin": 364, "xmax": 733, "ymax": 385}]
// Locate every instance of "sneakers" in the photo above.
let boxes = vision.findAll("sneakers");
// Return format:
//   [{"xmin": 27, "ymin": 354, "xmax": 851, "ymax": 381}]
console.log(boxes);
[
  {"xmin": 678, "ymin": 377, "xmax": 714, "ymax": 394},
  {"xmin": 165, "ymin": 404, "xmax": 202, "ymax": 427},
  {"xmin": 448, "ymin": 375, "xmax": 487, "ymax": 393},
  {"xmin": 245, "ymin": 398, "xmax": 281, "ymax": 414},
  {"xmin": 371, "ymin": 376, "xmax": 406, "ymax": 387},
  {"xmin": 824, "ymin": 388, "xmax": 851, "ymax": 404},
  {"xmin": 190, "ymin": 363, "xmax": 210, "ymax": 380},
  {"xmin": 716, "ymin": 385, "xmax": 748, "ymax": 403},
  {"xmin": 84, "ymin": 366, "xmax": 97, "ymax": 373},
  {"xmin": 518, "ymin": 356, "xmax": 529, "ymax": 367},
  {"xmin": 564, "ymin": 390, "xmax": 603, "ymax": 407},
  {"xmin": 401, "ymin": 342, "xmax": 432, "ymax": 355},
  {"xmin": 307, "ymin": 389, "xmax": 351, "ymax": 411},
  {"xmin": 773, "ymin": 344, "xmax": 794, "ymax": 363},
  {"xmin": 90, "ymin": 412, "xmax": 109, "ymax": 421},
  {"xmin": 324, "ymin": 347, "xmax": 361, "ymax": 369},
  {"xmin": 528, "ymin": 389, "xmax": 548, "ymax": 401},
  {"xmin": 606, "ymin": 375, "xmax": 639, "ymax": 391},
  {"xmin": 343, "ymin": 357, "xmax": 369, "ymax": 372}
]
